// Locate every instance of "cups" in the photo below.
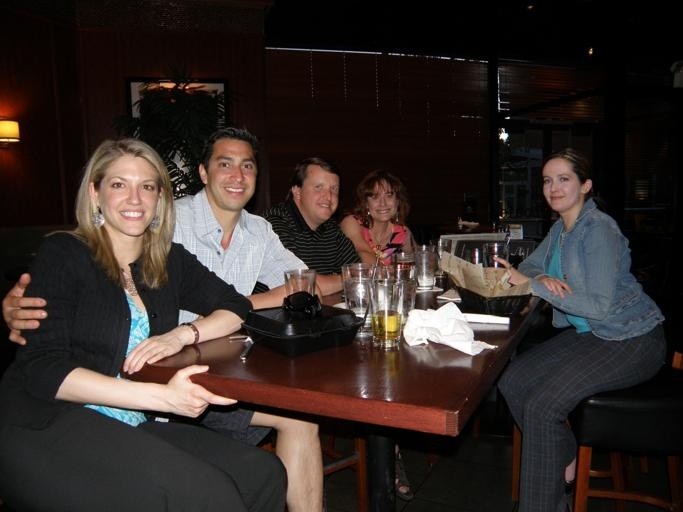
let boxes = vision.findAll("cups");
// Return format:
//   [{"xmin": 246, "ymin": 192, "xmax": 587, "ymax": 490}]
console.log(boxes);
[
  {"xmin": 341, "ymin": 236, "xmax": 453, "ymax": 350},
  {"xmin": 284, "ymin": 267, "xmax": 317, "ymax": 297},
  {"xmin": 472, "ymin": 242, "xmax": 511, "ymax": 266}
]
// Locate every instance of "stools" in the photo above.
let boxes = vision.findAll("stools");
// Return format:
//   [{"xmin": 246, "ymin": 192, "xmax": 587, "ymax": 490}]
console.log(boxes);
[
  {"xmin": 566, "ymin": 365, "xmax": 683, "ymax": 512},
  {"xmin": 260, "ymin": 430, "xmax": 367, "ymax": 511}
]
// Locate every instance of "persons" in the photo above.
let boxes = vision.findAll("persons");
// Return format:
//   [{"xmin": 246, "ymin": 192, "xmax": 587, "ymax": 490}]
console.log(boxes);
[
  {"xmin": 337, "ymin": 170, "xmax": 418, "ymax": 275},
  {"xmin": 0, "ymin": 137, "xmax": 287, "ymax": 511},
  {"xmin": 2, "ymin": 125, "xmax": 326, "ymax": 512},
  {"xmin": 492, "ymin": 148, "xmax": 668, "ymax": 512},
  {"xmin": 252, "ymin": 156, "xmax": 415, "ymax": 503}
]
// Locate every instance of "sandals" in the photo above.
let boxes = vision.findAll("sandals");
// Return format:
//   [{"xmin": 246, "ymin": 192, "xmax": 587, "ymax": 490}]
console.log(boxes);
[{"xmin": 393, "ymin": 448, "xmax": 414, "ymax": 502}]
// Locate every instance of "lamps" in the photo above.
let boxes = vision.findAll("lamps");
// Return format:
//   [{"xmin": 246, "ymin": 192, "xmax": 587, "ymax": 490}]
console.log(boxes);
[{"xmin": 0, "ymin": 121, "xmax": 20, "ymax": 142}]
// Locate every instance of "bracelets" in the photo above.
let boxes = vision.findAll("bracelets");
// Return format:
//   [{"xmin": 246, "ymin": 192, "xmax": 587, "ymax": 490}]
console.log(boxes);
[{"xmin": 181, "ymin": 321, "xmax": 201, "ymax": 345}]
